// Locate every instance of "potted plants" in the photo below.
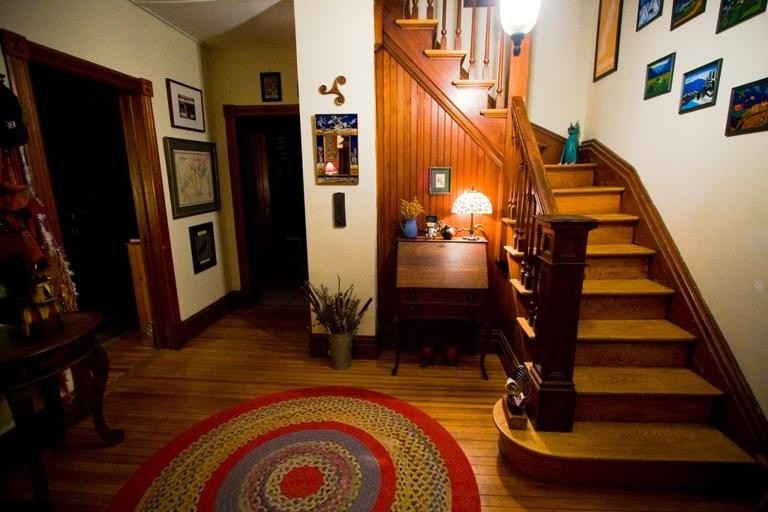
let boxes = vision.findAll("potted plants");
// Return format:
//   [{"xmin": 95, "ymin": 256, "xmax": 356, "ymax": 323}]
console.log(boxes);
[
  {"xmin": 299, "ymin": 272, "xmax": 376, "ymax": 370},
  {"xmin": 397, "ymin": 194, "xmax": 426, "ymax": 240}
]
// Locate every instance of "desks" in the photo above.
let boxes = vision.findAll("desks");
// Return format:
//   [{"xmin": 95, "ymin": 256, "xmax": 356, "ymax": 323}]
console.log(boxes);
[{"xmin": 0, "ymin": 309, "xmax": 127, "ymax": 512}]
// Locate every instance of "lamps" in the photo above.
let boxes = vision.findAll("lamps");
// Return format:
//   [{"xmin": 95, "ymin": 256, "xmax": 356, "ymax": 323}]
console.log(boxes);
[
  {"xmin": 495, "ymin": 1, "xmax": 543, "ymax": 58},
  {"xmin": 450, "ymin": 185, "xmax": 496, "ymax": 240}
]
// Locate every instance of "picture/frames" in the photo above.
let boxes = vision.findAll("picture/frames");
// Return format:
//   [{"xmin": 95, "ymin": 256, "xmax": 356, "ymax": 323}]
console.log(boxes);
[
  {"xmin": 162, "ymin": 76, "xmax": 207, "ymax": 134},
  {"xmin": 667, "ymin": 0, "xmax": 706, "ymax": 32},
  {"xmin": 713, "ymin": 0, "xmax": 767, "ymax": 36},
  {"xmin": 427, "ymin": 166, "xmax": 452, "ymax": 196},
  {"xmin": 643, "ymin": 51, "xmax": 677, "ymax": 101},
  {"xmin": 257, "ymin": 70, "xmax": 283, "ymax": 104},
  {"xmin": 677, "ymin": 57, "xmax": 725, "ymax": 114},
  {"xmin": 724, "ymin": 76, "xmax": 767, "ymax": 138},
  {"xmin": 633, "ymin": 0, "xmax": 664, "ymax": 33},
  {"xmin": 590, "ymin": 0, "xmax": 624, "ymax": 85},
  {"xmin": 161, "ymin": 136, "xmax": 225, "ymax": 221},
  {"xmin": 187, "ymin": 220, "xmax": 218, "ymax": 277}
]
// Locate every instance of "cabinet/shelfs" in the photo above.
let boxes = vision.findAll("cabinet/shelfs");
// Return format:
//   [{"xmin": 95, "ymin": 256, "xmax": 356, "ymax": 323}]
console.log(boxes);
[{"xmin": 389, "ymin": 235, "xmax": 491, "ymax": 380}]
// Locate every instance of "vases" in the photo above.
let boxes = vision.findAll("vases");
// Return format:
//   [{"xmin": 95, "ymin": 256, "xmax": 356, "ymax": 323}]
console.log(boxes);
[{"xmin": 561, "ymin": 127, "xmax": 581, "ymax": 165}]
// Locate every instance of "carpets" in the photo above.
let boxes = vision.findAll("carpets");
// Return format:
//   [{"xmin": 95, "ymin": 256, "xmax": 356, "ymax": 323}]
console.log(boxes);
[{"xmin": 106, "ymin": 382, "xmax": 482, "ymax": 511}]
